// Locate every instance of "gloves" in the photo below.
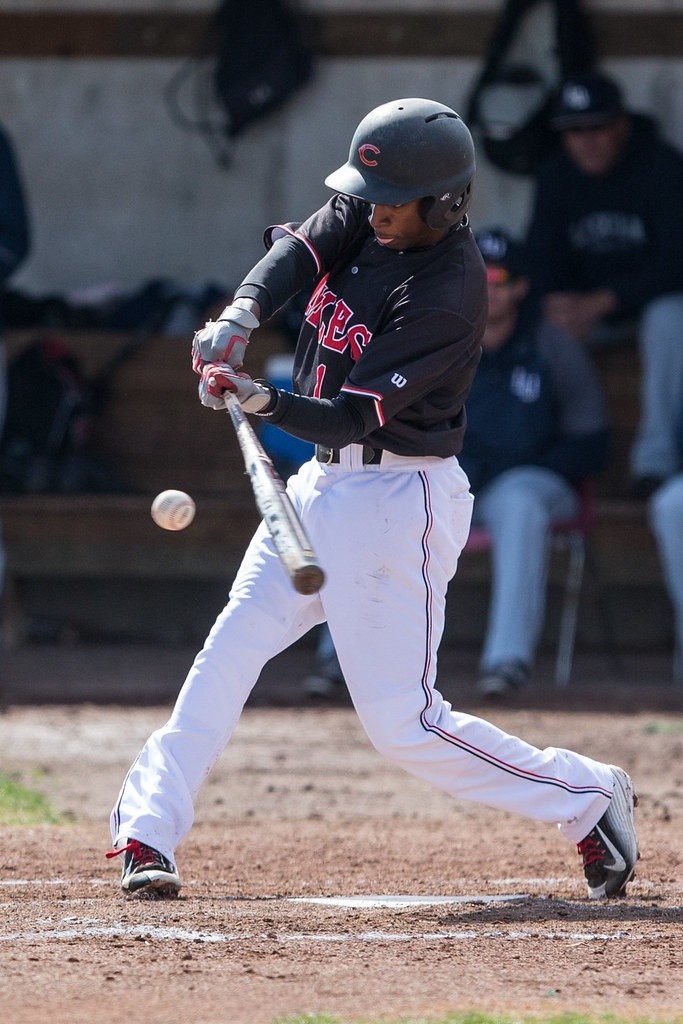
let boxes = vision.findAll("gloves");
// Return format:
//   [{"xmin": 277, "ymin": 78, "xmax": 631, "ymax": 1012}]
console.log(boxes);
[
  {"xmin": 192, "ymin": 307, "xmax": 258, "ymax": 376},
  {"xmin": 198, "ymin": 363, "xmax": 272, "ymax": 415}
]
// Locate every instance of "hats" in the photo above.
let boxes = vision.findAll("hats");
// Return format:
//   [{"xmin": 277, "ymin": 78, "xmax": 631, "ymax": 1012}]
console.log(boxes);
[
  {"xmin": 475, "ymin": 228, "xmax": 531, "ymax": 279},
  {"xmin": 555, "ymin": 73, "xmax": 621, "ymax": 130}
]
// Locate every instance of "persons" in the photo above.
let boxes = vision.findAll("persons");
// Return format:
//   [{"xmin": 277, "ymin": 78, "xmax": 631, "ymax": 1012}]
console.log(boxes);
[
  {"xmin": 301, "ymin": 229, "xmax": 607, "ymax": 703},
  {"xmin": 109, "ymin": 97, "xmax": 641, "ymax": 901},
  {"xmin": 521, "ymin": 71, "xmax": 683, "ymax": 499}
]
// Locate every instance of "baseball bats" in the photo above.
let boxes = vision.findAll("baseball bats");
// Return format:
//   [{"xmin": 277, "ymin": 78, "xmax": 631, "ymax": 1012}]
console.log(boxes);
[{"xmin": 222, "ymin": 390, "xmax": 325, "ymax": 597}]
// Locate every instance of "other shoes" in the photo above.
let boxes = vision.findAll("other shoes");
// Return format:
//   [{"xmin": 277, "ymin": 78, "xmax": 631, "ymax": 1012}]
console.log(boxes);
[
  {"xmin": 481, "ymin": 661, "xmax": 522, "ymax": 696},
  {"xmin": 307, "ymin": 664, "xmax": 340, "ymax": 697}
]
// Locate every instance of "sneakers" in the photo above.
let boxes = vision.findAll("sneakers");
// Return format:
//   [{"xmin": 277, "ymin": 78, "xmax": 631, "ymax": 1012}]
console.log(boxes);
[
  {"xmin": 107, "ymin": 841, "xmax": 184, "ymax": 897},
  {"xmin": 578, "ymin": 765, "xmax": 640, "ymax": 899}
]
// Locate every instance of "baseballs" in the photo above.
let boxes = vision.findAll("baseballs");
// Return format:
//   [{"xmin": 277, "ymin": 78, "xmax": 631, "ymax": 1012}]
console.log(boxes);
[{"xmin": 150, "ymin": 489, "xmax": 197, "ymax": 532}]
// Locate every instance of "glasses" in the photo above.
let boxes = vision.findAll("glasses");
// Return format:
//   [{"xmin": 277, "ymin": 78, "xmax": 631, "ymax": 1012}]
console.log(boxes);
[{"xmin": 485, "ymin": 269, "xmax": 515, "ymax": 287}]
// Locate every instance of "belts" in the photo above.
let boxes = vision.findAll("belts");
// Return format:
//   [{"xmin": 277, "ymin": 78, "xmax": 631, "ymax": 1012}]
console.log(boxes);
[{"xmin": 315, "ymin": 441, "xmax": 380, "ymax": 465}]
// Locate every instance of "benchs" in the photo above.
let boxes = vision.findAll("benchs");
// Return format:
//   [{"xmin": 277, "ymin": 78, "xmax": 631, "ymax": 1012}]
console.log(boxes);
[{"xmin": 0, "ymin": 321, "xmax": 662, "ymax": 689}]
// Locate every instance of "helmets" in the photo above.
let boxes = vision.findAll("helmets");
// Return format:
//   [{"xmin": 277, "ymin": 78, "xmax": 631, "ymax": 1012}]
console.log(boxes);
[{"xmin": 323, "ymin": 97, "xmax": 474, "ymax": 228}]
